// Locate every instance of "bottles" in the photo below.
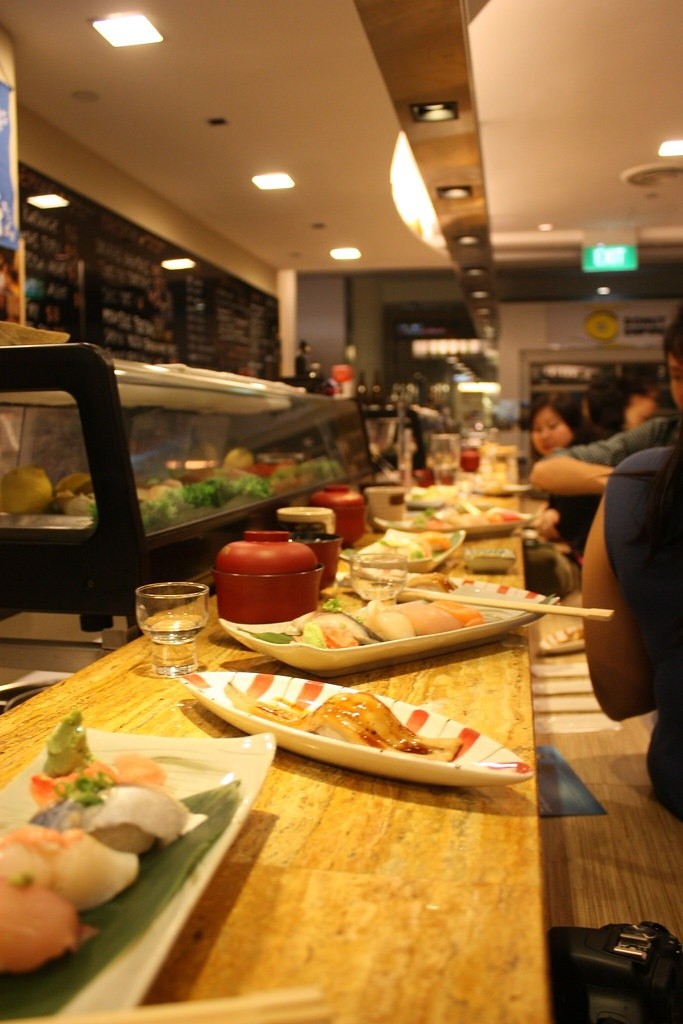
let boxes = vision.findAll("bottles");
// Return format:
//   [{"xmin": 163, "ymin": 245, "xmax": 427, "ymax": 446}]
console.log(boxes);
[
  {"xmin": 522, "ymin": 525, "xmax": 565, "ymax": 602},
  {"xmin": 309, "ymin": 363, "xmax": 322, "ymax": 393}
]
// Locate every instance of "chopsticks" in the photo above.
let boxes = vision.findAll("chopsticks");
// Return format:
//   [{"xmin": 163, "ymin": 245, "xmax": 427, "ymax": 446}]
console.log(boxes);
[
  {"xmin": 399, "ymin": 586, "xmax": 615, "ymax": 622},
  {"xmin": 0, "ymin": 985, "xmax": 332, "ymax": 1024}
]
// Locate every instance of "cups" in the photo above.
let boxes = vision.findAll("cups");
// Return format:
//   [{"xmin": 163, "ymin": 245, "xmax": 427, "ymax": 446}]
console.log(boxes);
[
  {"xmin": 350, "ymin": 553, "xmax": 408, "ymax": 606},
  {"xmin": 136, "ymin": 581, "xmax": 209, "ymax": 677},
  {"xmin": 277, "ymin": 507, "xmax": 335, "ymax": 535},
  {"xmin": 365, "ymin": 487, "xmax": 405, "ymax": 532},
  {"xmin": 430, "ymin": 433, "xmax": 461, "ymax": 485},
  {"xmin": 439, "ymin": 470, "xmax": 455, "ymax": 487},
  {"xmin": 331, "ymin": 364, "xmax": 354, "ymax": 400},
  {"xmin": 462, "ymin": 448, "xmax": 480, "ymax": 472}
]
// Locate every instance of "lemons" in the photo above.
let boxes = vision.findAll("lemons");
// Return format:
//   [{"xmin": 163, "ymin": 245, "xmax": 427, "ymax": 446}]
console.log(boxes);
[
  {"xmin": 53, "ymin": 471, "xmax": 91, "ymax": 492},
  {"xmin": 223, "ymin": 447, "xmax": 253, "ymax": 470},
  {"xmin": 0, "ymin": 463, "xmax": 55, "ymax": 516}
]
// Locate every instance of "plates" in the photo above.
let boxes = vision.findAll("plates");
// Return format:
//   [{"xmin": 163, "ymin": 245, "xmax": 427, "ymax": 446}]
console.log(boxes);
[
  {"xmin": 542, "ymin": 626, "xmax": 587, "ymax": 653},
  {"xmin": 464, "ymin": 548, "xmax": 516, "ymax": 572},
  {"xmin": 352, "ymin": 528, "xmax": 466, "ymax": 572},
  {"xmin": 0, "ymin": 728, "xmax": 276, "ymax": 1024},
  {"xmin": 182, "ymin": 673, "xmax": 534, "ymax": 787},
  {"xmin": 472, "ymin": 479, "xmax": 534, "ymax": 496},
  {"xmin": 375, "ymin": 507, "xmax": 533, "ymax": 536},
  {"xmin": 406, "ymin": 484, "xmax": 461, "ymax": 510},
  {"xmin": 218, "ymin": 578, "xmax": 560, "ymax": 674}
]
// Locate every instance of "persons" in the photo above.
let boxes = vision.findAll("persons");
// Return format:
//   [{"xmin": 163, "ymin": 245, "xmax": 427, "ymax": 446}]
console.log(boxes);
[{"xmin": 526, "ymin": 305, "xmax": 683, "ymax": 823}]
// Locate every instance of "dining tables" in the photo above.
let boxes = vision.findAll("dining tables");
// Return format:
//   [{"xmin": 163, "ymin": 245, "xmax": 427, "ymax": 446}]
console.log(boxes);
[
  {"xmin": 0, "ymin": 460, "xmax": 552, "ymax": 1024},
  {"xmin": 530, "ymin": 592, "xmax": 683, "ymax": 1023}
]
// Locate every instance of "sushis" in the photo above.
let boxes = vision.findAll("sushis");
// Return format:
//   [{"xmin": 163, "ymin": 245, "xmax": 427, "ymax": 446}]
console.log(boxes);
[{"xmin": 0, "ymin": 507, "xmax": 464, "ymax": 977}]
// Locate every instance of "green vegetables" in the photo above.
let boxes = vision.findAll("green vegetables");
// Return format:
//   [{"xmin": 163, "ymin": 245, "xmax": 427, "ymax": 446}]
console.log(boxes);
[{"xmin": 89, "ymin": 472, "xmax": 273, "ymax": 529}]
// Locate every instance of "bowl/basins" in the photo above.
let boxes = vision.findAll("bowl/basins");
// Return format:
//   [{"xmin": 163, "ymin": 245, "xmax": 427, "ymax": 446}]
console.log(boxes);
[
  {"xmin": 414, "ymin": 468, "xmax": 433, "ymax": 488},
  {"xmin": 289, "ymin": 531, "xmax": 343, "ymax": 590},
  {"xmin": 211, "ymin": 529, "xmax": 324, "ymax": 623},
  {"xmin": 311, "ymin": 485, "xmax": 367, "ymax": 548}
]
